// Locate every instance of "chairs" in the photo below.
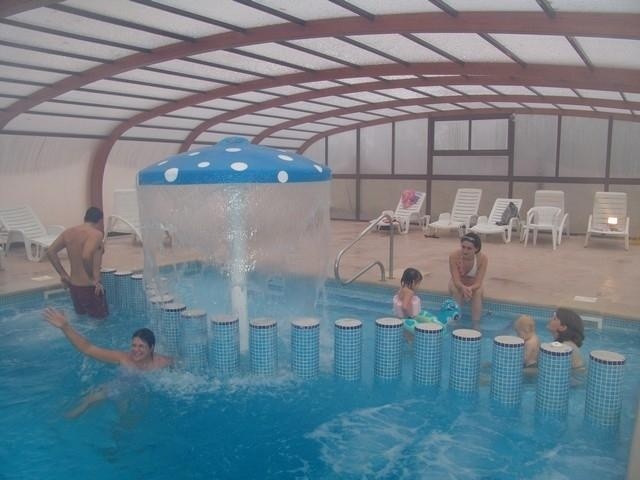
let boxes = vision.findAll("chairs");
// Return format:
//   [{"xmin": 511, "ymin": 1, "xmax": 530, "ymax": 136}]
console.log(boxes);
[
  {"xmin": 0, "ymin": 204, "xmax": 71, "ymax": 263},
  {"xmin": 102, "ymin": 188, "xmax": 174, "ymax": 248},
  {"xmin": 427, "ymin": 187, "xmax": 484, "ymax": 241},
  {"xmin": 524, "ymin": 189, "xmax": 570, "ymax": 246},
  {"xmin": 525, "ymin": 205, "xmax": 563, "ymax": 251},
  {"xmin": 469, "ymin": 197, "xmax": 525, "ymax": 244},
  {"xmin": 373, "ymin": 189, "xmax": 431, "ymax": 235},
  {"xmin": 582, "ymin": 191, "xmax": 632, "ymax": 252}
]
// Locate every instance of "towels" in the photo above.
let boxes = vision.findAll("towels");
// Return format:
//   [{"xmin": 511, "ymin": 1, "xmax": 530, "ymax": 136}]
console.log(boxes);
[
  {"xmin": 496, "ymin": 202, "xmax": 521, "ymax": 226},
  {"xmin": 400, "ymin": 190, "xmax": 418, "ymax": 208}
]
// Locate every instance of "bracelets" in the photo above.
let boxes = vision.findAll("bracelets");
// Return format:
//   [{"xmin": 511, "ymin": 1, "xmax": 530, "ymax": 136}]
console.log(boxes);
[{"xmin": 95, "ymin": 281, "xmax": 101, "ymax": 286}]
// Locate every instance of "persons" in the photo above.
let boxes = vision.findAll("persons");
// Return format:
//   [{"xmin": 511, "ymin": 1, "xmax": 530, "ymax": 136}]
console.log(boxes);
[
  {"xmin": 45, "ymin": 207, "xmax": 110, "ymax": 319},
  {"xmin": 476, "ymin": 308, "xmax": 590, "ymax": 388},
  {"xmin": 391, "ymin": 268, "xmax": 424, "ymax": 349},
  {"xmin": 40, "ymin": 306, "xmax": 176, "ymax": 431},
  {"xmin": 446, "ymin": 232, "xmax": 488, "ymax": 336},
  {"xmin": 512, "ymin": 315, "xmax": 541, "ymax": 369}
]
